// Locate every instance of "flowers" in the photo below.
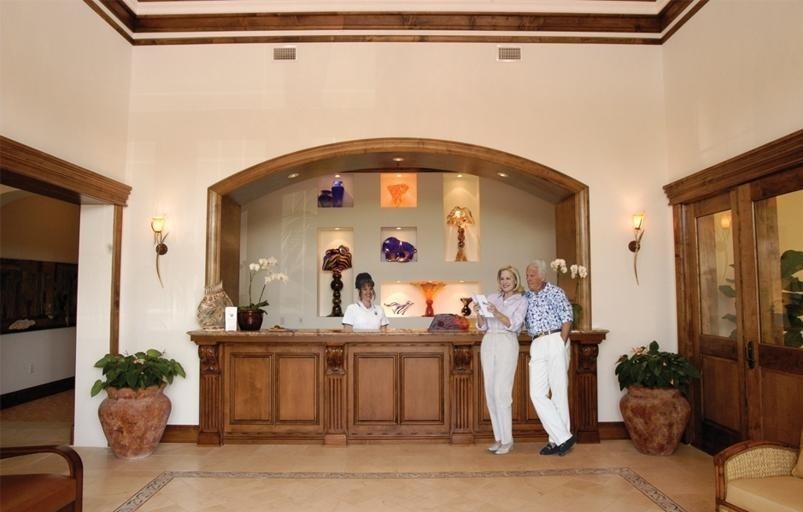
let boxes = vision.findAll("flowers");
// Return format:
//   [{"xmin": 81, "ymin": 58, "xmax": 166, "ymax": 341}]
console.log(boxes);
[
  {"xmin": 548, "ymin": 257, "xmax": 590, "ymax": 323},
  {"xmin": 613, "ymin": 336, "xmax": 702, "ymax": 391},
  {"xmin": 85, "ymin": 344, "xmax": 188, "ymax": 398},
  {"xmin": 239, "ymin": 258, "xmax": 291, "ymax": 308}
]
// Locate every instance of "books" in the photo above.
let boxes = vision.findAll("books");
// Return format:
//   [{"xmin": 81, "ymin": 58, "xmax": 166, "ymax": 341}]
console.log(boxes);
[{"xmin": 471, "ymin": 293, "xmax": 496, "ymax": 319}]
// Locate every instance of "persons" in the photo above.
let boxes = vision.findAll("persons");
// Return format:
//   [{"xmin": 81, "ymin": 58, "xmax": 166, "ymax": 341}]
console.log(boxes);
[
  {"xmin": 521, "ymin": 258, "xmax": 577, "ymax": 456},
  {"xmin": 475, "ymin": 263, "xmax": 530, "ymax": 454},
  {"xmin": 340, "ymin": 272, "xmax": 390, "ymax": 334}
]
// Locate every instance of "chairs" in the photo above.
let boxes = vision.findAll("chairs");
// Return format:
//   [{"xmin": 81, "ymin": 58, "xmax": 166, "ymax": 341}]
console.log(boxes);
[
  {"xmin": 1, "ymin": 444, "xmax": 86, "ymax": 510},
  {"xmin": 713, "ymin": 439, "xmax": 803, "ymax": 512}
]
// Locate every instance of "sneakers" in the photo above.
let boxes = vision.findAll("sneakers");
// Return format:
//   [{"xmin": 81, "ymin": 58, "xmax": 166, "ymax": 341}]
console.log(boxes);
[
  {"xmin": 559, "ymin": 435, "xmax": 576, "ymax": 456},
  {"xmin": 540, "ymin": 444, "xmax": 559, "ymax": 455}
]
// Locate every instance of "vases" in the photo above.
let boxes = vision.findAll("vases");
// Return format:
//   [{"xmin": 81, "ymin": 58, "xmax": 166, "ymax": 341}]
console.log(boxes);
[
  {"xmin": 620, "ymin": 386, "xmax": 693, "ymax": 457},
  {"xmin": 97, "ymin": 384, "xmax": 173, "ymax": 461},
  {"xmin": 232, "ymin": 303, "xmax": 272, "ymax": 332}
]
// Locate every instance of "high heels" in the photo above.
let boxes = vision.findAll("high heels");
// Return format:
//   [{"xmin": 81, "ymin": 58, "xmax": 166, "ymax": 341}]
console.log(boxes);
[{"xmin": 495, "ymin": 441, "xmax": 514, "ymax": 454}]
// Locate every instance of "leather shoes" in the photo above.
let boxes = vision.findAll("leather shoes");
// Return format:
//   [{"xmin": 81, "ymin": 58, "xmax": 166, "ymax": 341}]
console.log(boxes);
[{"xmin": 488, "ymin": 445, "xmax": 500, "ymax": 452}]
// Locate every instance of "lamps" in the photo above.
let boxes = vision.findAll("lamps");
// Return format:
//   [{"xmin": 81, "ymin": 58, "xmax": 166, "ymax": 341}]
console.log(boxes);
[
  {"xmin": 148, "ymin": 214, "xmax": 183, "ymax": 293},
  {"xmin": 714, "ymin": 212, "xmax": 734, "ymax": 282},
  {"xmin": 623, "ymin": 212, "xmax": 658, "ymax": 288},
  {"xmin": 447, "ymin": 204, "xmax": 476, "ymax": 264}
]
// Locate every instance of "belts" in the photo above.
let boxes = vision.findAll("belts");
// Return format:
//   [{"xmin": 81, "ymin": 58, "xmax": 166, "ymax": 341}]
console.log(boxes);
[{"xmin": 533, "ymin": 329, "xmax": 561, "ymax": 338}]
